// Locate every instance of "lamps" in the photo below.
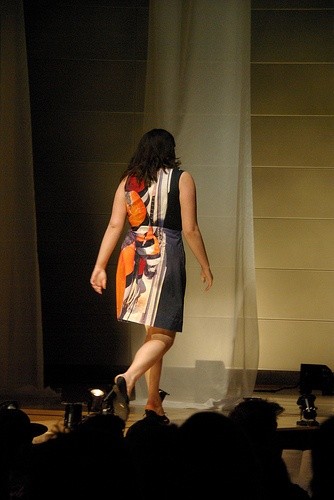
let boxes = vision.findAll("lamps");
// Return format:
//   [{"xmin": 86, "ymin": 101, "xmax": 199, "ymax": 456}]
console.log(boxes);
[
  {"xmin": 64, "ymin": 404, "xmax": 85, "ymax": 433},
  {"xmin": 87, "ymin": 386, "xmax": 107, "ymax": 416},
  {"xmin": 296, "ymin": 394, "xmax": 320, "ymax": 426}
]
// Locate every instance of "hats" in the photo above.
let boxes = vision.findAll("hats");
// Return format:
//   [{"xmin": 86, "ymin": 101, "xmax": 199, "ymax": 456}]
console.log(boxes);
[{"xmin": 0, "ymin": 408, "xmax": 47, "ymax": 440}]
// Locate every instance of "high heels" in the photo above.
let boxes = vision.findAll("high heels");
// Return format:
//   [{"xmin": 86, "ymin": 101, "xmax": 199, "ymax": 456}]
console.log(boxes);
[
  {"xmin": 145, "ymin": 408, "xmax": 170, "ymax": 424},
  {"xmin": 104, "ymin": 375, "xmax": 129, "ymax": 410}
]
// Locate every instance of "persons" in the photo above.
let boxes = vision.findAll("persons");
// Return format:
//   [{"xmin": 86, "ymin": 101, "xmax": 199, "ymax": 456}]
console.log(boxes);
[
  {"xmin": 0, "ymin": 400, "xmax": 334, "ymax": 500},
  {"xmin": 90, "ymin": 129, "xmax": 213, "ymax": 422}
]
